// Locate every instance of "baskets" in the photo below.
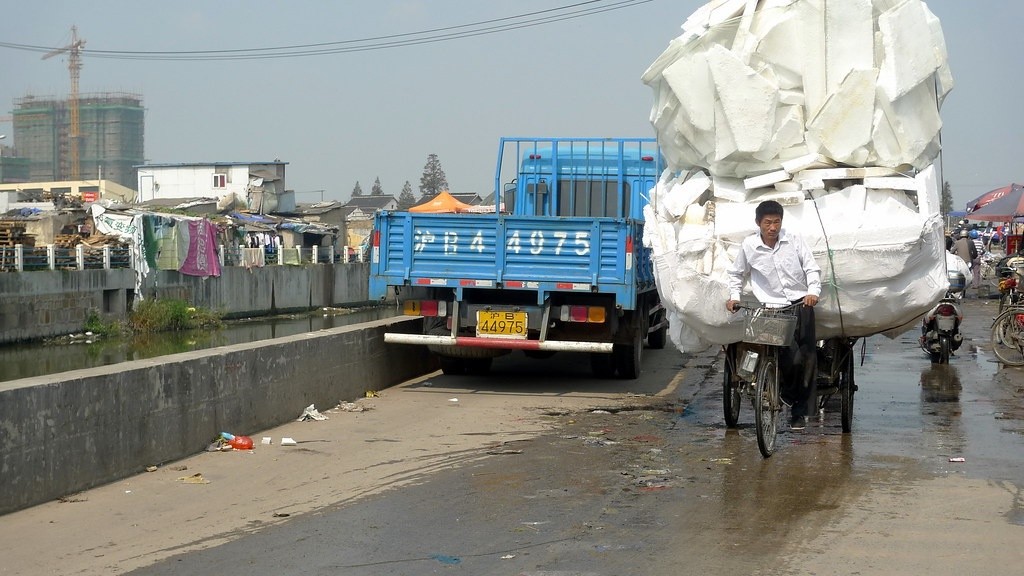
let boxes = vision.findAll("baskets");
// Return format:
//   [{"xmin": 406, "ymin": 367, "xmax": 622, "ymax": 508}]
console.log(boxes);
[
  {"xmin": 740, "ymin": 307, "xmax": 798, "ymax": 347},
  {"xmin": 1017, "ymin": 275, "xmax": 1024, "ymax": 293}
]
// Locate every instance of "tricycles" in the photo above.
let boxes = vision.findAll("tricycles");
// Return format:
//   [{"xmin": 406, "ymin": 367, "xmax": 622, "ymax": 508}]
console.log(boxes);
[
  {"xmin": 988, "ymin": 264, "xmax": 1024, "ymax": 370},
  {"xmin": 723, "ymin": 299, "xmax": 860, "ymax": 457}
]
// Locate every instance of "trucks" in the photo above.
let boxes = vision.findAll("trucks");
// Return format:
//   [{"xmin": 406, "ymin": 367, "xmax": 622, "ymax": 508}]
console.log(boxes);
[{"xmin": 367, "ymin": 136, "xmax": 682, "ymax": 382}]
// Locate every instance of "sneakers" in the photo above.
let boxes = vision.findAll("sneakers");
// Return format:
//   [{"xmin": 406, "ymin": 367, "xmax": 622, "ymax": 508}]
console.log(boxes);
[
  {"xmin": 781, "ymin": 391, "xmax": 794, "ymax": 408},
  {"xmin": 790, "ymin": 415, "xmax": 806, "ymax": 429}
]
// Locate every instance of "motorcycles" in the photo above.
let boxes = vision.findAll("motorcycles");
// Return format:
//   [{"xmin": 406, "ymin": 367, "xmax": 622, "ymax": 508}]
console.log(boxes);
[{"xmin": 919, "ymin": 270, "xmax": 965, "ymax": 367}]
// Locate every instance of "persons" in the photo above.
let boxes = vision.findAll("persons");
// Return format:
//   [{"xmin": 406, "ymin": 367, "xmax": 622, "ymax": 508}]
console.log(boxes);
[
  {"xmin": 722, "ymin": 201, "xmax": 823, "ymax": 431},
  {"xmin": 951, "ymin": 219, "xmax": 997, "ymax": 260},
  {"xmin": 942, "ymin": 237, "xmax": 972, "ymax": 289}
]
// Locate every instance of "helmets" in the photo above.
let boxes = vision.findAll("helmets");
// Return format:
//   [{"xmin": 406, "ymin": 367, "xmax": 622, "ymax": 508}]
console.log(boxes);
[
  {"xmin": 964, "ymin": 219, "xmax": 969, "ymax": 223},
  {"xmin": 960, "ymin": 229, "xmax": 968, "ymax": 237},
  {"xmin": 959, "ymin": 220, "xmax": 964, "ymax": 226},
  {"xmin": 969, "ymin": 229, "xmax": 978, "ymax": 238}
]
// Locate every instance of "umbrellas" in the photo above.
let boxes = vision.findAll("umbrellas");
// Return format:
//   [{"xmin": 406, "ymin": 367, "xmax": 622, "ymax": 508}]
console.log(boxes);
[{"xmin": 947, "ymin": 184, "xmax": 1024, "ymax": 224}]
